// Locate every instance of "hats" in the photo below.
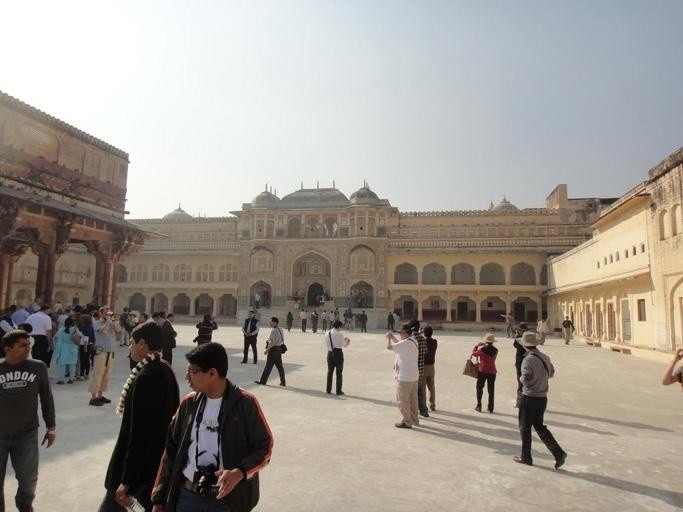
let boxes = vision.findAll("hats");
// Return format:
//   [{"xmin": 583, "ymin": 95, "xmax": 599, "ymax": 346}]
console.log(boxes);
[
  {"xmin": 392, "ymin": 325, "xmax": 412, "ymax": 333},
  {"xmin": 483, "ymin": 333, "xmax": 498, "ymax": 343},
  {"xmin": 515, "ymin": 331, "xmax": 542, "ymax": 347}
]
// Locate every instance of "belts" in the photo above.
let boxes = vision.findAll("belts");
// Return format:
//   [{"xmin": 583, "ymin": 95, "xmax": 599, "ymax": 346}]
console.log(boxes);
[{"xmin": 181, "ymin": 479, "xmax": 220, "ymax": 495}]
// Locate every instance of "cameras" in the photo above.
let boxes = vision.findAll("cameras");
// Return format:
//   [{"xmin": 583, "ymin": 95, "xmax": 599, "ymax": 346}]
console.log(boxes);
[
  {"xmin": 193, "ymin": 336, "xmax": 198, "ymax": 343},
  {"xmin": 193, "ymin": 465, "xmax": 218, "ymax": 499},
  {"xmin": 266, "ymin": 340, "xmax": 270, "ymax": 349},
  {"xmin": 210, "ymin": 318, "xmax": 214, "ymax": 321},
  {"xmin": 105, "ymin": 312, "xmax": 117, "ymax": 316}
]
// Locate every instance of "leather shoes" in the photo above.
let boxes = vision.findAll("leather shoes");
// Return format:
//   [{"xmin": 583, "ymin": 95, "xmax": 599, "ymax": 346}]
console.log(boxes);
[
  {"xmin": 555, "ymin": 451, "xmax": 567, "ymax": 469},
  {"xmin": 513, "ymin": 456, "xmax": 532, "ymax": 465},
  {"xmin": 254, "ymin": 381, "xmax": 266, "ymax": 386},
  {"xmin": 327, "ymin": 389, "xmax": 344, "ymax": 396}
]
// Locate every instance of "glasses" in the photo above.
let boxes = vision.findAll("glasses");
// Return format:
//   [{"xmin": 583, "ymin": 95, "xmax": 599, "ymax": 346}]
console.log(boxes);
[{"xmin": 186, "ymin": 368, "xmax": 205, "ymax": 375}]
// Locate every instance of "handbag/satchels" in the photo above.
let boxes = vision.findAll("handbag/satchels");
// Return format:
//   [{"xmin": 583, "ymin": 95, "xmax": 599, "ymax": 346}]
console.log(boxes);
[
  {"xmin": 462, "ymin": 353, "xmax": 481, "ymax": 378},
  {"xmin": 281, "ymin": 343, "xmax": 288, "ymax": 355},
  {"xmin": 333, "ymin": 348, "xmax": 343, "ymax": 363},
  {"xmin": 72, "ymin": 335, "xmax": 83, "ymax": 345}
]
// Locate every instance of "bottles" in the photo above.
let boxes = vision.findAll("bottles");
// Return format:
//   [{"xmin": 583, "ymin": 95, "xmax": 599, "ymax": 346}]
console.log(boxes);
[{"xmin": 124, "ymin": 496, "xmax": 145, "ymax": 512}]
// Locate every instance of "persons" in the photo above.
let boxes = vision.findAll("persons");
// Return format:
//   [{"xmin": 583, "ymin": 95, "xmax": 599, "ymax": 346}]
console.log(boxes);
[
  {"xmin": 87, "ymin": 305, "xmax": 122, "ymax": 406},
  {"xmin": 293, "ymin": 286, "xmax": 403, "ymax": 334},
  {"xmin": 149, "ymin": 342, "xmax": 274, "ymax": 512},
  {"xmin": 98, "ymin": 319, "xmax": 181, "ymax": 512},
  {"xmin": 511, "ymin": 329, "xmax": 566, "ymax": 470},
  {"xmin": 512, "ymin": 321, "xmax": 530, "ymax": 409},
  {"xmin": 385, "ymin": 325, "xmax": 420, "ymax": 429},
  {"xmin": 253, "ymin": 317, "xmax": 287, "ymax": 387},
  {"xmin": 324, "ymin": 320, "xmax": 350, "ymax": 395},
  {"xmin": 471, "ymin": 338, "xmax": 498, "ymax": 414},
  {"xmin": 0, "ymin": 328, "xmax": 59, "ymax": 512},
  {"xmin": 500, "ymin": 312, "xmax": 514, "ymax": 339},
  {"xmin": 285, "ymin": 311, "xmax": 293, "ymax": 332},
  {"xmin": 0, "ymin": 295, "xmax": 176, "ymax": 384},
  {"xmin": 661, "ymin": 347, "xmax": 682, "ymax": 399},
  {"xmin": 561, "ymin": 316, "xmax": 574, "ymax": 346},
  {"xmin": 254, "ymin": 291, "xmax": 261, "ymax": 309},
  {"xmin": 420, "ymin": 324, "xmax": 437, "ymax": 412},
  {"xmin": 406, "ymin": 318, "xmax": 429, "ymax": 419},
  {"xmin": 536, "ymin": 317, "xmax": 547, "ymax": 345},
  {"xmin": 195, "ymin": 314, "xmax": 218, "ymax": 347},
  {"xmin": 240, "ymin": 311, "xmax": 259, "ymax": 364}
]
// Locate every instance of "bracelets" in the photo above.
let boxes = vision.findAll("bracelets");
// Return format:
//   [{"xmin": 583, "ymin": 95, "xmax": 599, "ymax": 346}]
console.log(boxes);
[{"xmin": 47, "ymin": 430, "xmax": 56, "ymax": 436}]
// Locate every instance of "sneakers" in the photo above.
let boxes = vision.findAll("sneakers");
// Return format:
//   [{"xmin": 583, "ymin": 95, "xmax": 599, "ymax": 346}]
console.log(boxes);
[
  {"xmin": 100, "ymin": 397, "xmax": 112, "ymax": 404},
  {"xmin": 89, "ymin": 398, "xmax": 104, "ymax": 406},
  {"xmin": 394, "ymin": 422, "xmax": 412, "ymax": 428}
]
